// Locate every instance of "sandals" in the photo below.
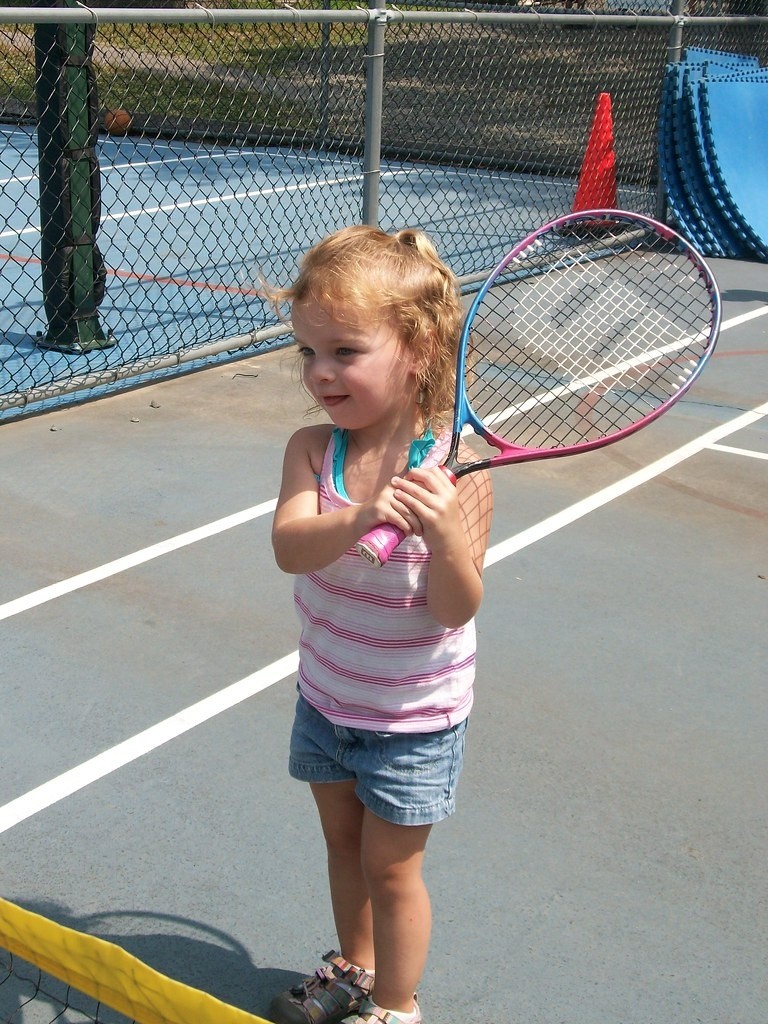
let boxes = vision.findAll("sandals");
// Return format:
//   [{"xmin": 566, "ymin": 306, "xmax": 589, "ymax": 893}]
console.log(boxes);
[
  {"xmin": 336, "ymin": 992, "xmax": 420, "ymax": 1024},
  {"xmin": 269, "ymin": 950, "xmax": 376, "ymax": 1024}
]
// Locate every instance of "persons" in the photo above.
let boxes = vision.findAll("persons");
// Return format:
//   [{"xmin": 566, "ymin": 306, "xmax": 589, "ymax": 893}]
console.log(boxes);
[{"xmin": 239, "ymin": 226, "xmax": 494, "ymax": 1024}]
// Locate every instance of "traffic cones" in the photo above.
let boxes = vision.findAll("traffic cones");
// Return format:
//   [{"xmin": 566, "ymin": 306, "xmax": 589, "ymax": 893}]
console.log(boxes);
[{"xmin": 550, "ymin": 90, "xmax": 630, "ymax": 240}]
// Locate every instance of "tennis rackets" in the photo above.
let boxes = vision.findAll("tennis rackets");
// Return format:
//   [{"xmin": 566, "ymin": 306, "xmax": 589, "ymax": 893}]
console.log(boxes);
[{"xmin": 354, "ymin": 207, "xmax": 727, "ymax": 571}]
